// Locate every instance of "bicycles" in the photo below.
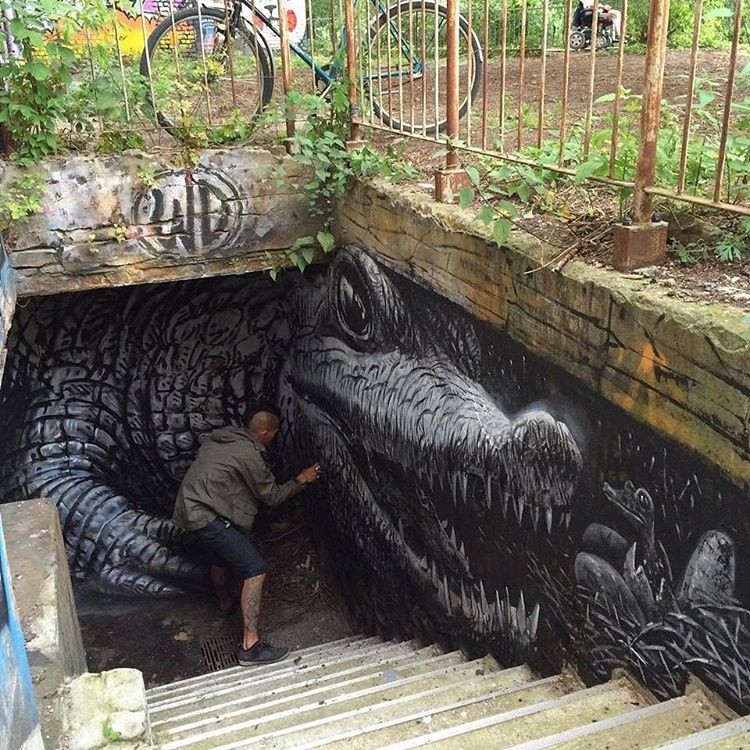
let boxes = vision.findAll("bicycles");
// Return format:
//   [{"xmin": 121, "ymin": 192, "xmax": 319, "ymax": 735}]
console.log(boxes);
[{"xmin": 137, "ymin": 0, "xmax": 486, "ymax": 148}]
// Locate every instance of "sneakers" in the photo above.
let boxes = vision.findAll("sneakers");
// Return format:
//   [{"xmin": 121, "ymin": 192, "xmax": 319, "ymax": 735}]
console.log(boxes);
[
  {"xmin": 238, "ymin": 638, "xmax": 291, "ymax": 666},
  {"xmin": 215, "ymin": 598, "xmax": 240, "ymax": 620}
]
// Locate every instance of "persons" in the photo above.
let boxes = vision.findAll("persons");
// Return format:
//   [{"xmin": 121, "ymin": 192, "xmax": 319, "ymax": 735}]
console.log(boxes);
[
  {"xmin": 170, "ymin": 411, "xmax": 324, "ymax": 665},
  {"xmin": 574, "ymin": 0, "xmax": 628, "ymax": 45}
]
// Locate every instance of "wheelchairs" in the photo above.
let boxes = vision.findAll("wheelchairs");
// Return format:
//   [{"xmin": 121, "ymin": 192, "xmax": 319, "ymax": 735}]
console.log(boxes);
[{"xmin": 567, "ymin": 6, "xmax": 625, "ymax": 51}]
